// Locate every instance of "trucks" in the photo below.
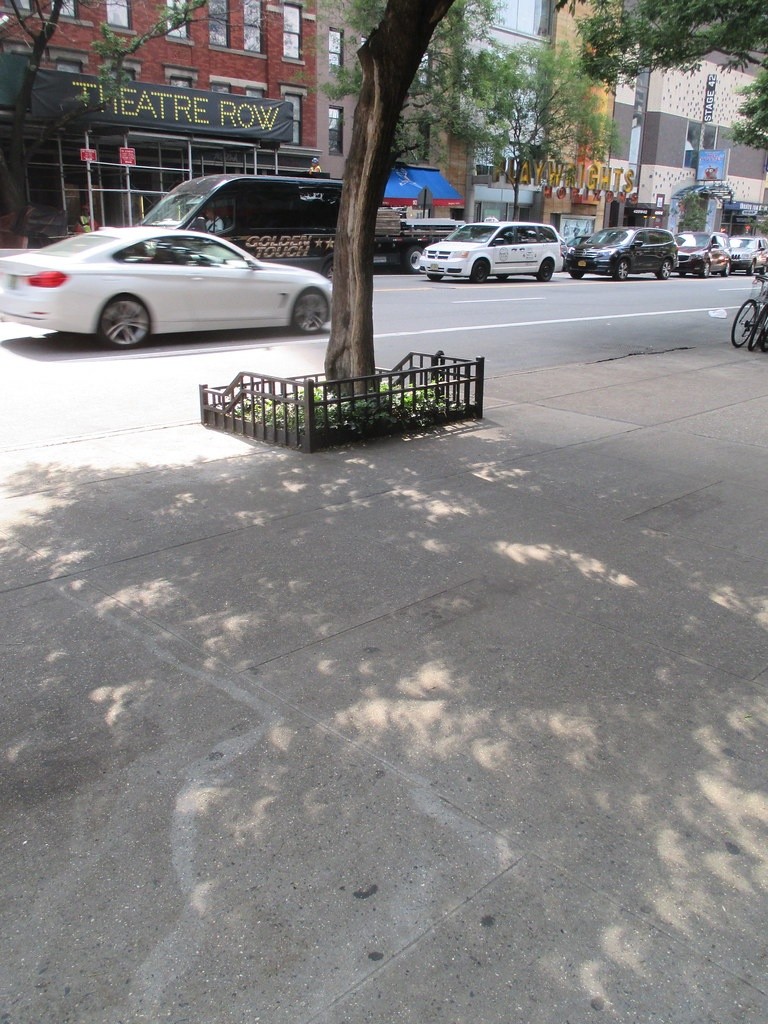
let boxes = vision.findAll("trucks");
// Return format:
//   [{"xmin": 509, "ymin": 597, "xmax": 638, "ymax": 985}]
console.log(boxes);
[{"xmin": 134, "ymin": 172, "xmax": 456, "ymax": 283}]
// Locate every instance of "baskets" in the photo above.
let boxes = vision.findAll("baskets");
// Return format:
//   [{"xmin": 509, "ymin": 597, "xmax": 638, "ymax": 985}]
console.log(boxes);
[{"xmin": 749, "ymin": 279, "xmax": 768, "ymax": 304}]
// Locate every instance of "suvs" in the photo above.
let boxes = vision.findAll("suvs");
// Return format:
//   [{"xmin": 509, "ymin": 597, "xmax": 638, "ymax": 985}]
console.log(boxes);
[
  {"xmin": 729, "ymin": 234, "xmax": 768, "ymax": 276},
  {"xmin": 416, "ymin": 220, "xmax": 565, "ymax": 284},
  {"xmin": 564, "ymin": 224, "xmax": 679, "ymax": 281},
  {"xmin": 671, "ymin": 231, "xmax": 733, "ymax": 278}
]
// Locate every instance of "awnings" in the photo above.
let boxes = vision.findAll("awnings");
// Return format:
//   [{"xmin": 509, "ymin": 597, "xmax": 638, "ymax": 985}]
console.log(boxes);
[{"xmin": 382, "ymin": 167, "xmax": 465, "ymax": 208}]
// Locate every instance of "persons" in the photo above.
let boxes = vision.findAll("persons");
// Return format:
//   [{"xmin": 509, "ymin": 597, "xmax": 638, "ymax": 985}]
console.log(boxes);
[
  {"xmin": 205, "ymin": 206, "xmax": 223, "ymax": 233},
  {"xmin": 309, "ymin": 158, "xmax": 321, "ymax": 176},
  {"xmin": 720, "ymin": 225, "xmax": 751, "ymax": 236},
  {"xmin": 74, "ymin": 205, "xmax": 98, "ymax": 232}
]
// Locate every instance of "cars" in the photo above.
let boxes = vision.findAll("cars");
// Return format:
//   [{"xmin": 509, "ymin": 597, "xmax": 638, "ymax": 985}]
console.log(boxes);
[
  {"xmin": 1, "ymin": 224, "xmax": 333, "ymax": 350},
  {"xmin": 565, "ymin": 234, "xmax": 605, "ymax": 267}
]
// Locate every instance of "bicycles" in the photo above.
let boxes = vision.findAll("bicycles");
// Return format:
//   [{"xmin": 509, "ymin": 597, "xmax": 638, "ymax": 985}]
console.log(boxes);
[{"xmin": 731, "ymin": 268, "xmax": 767, "ymax": 353}]
